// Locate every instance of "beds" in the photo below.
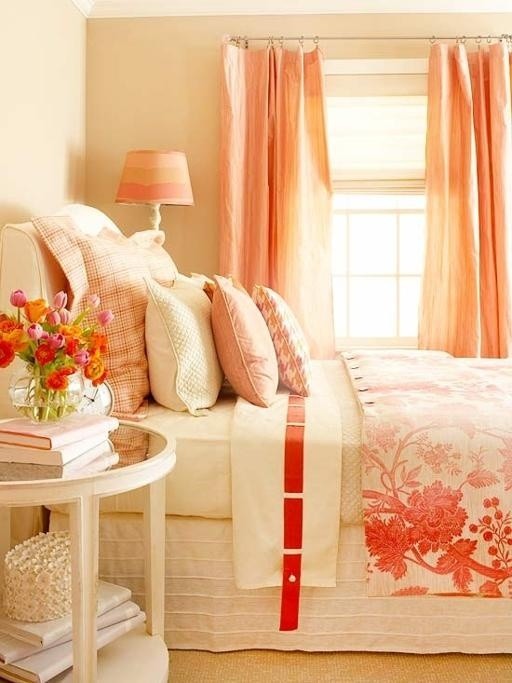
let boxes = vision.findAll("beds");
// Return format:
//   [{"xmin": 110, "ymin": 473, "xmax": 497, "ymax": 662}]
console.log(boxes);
[{"xmin": 0, "ymin": 198, "xmax": 511, "ymax": 657}]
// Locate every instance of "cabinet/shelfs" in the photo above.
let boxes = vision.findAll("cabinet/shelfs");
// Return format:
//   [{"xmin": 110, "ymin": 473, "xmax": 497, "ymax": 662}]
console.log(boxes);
[{"xmin": 0, "ymin": 420, "xmax": 180, "ymax": 682}]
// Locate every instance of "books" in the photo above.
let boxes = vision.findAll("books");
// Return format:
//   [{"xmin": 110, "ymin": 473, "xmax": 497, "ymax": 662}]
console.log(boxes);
[
  {"xmin": 1, "ymin": 414, "xmax": 123, "ymax": 482},
  {"xmin": 0, "ymin": 579, "xmax": 148, "ymax": 683}
]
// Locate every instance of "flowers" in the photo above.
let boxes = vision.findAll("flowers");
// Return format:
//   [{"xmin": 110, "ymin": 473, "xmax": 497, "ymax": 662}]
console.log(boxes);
[{"xmin": 0, "ymin": 281, "xmax": 118, "ymax": 408}]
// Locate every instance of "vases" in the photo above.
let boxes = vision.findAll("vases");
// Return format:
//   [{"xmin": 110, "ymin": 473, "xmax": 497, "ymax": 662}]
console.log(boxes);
[{"xmin": 8, "ymin": 366, "xmax": 91, "ymax": 423}]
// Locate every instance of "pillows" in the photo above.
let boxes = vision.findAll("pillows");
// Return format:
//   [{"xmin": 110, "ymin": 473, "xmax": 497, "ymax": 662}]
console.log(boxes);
[
  {"xmin": 175, "ymin": 265, "xmax": 213, "ymax": 289},
  {"xmin": 139, "ymin": 268, "xmax": 222, "ymax": 416},
  {"xmin": 26, "ymin": 202, "xmax": 179, "ymax": 419},
  {"xmin": 208, "ymin": 269, "xmax": 279, "ymax": 408},
  {"xmin": 248, "ymin": 277, "xmax": 315, "ymax": 399}
]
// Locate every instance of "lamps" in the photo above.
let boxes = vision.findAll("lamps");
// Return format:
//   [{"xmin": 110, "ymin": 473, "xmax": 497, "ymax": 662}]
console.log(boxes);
[{"xmin": 114, "ymin": 142, "xmax": 197, "ymax": 232}]
